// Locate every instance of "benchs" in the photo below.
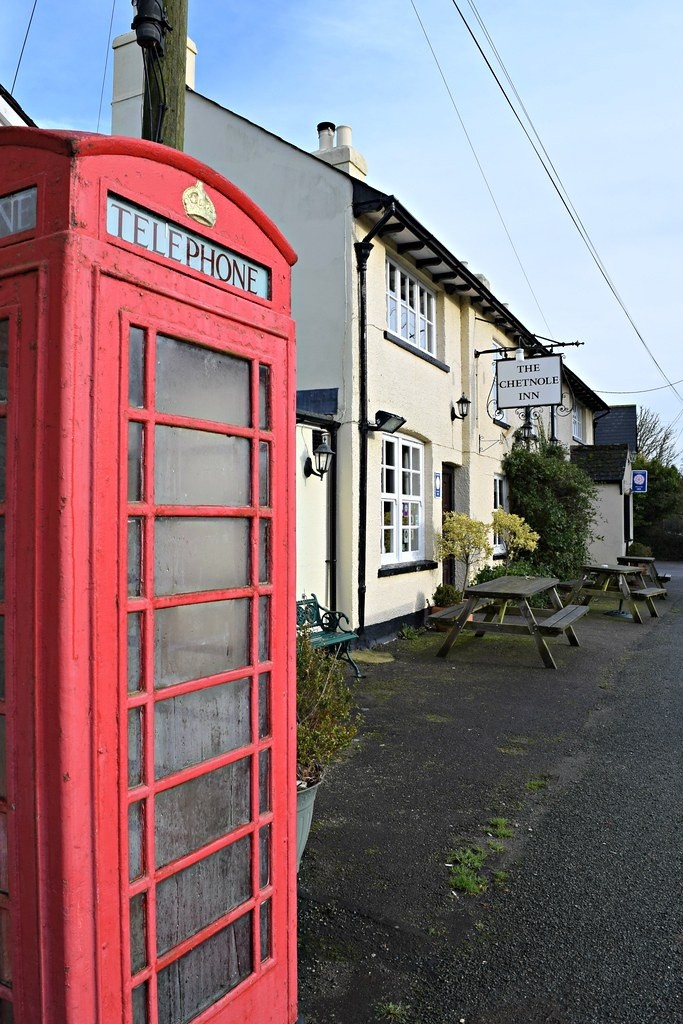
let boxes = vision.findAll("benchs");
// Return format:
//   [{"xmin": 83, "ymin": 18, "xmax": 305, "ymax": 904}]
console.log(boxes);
[
  {"xmin": 428, "ymin": 598, "xmax": 494, "ymax": 627},
  {"xmin": 296, "ymin": 594, "xmax": 362, "ymax": 679},
  {"xmin": 557, "ymin": 580, "xmax": 596, "ymax": 593},
  {"xmin": 536, "ymin": 604, "xmax": 590, "ymax": 637},
  {"xmin": 631, "ymin": 587, "xmax": 667, "ymax": 600},
  {"xmin": 657, "ymin": 572, "xmax": 671, "ymax": 583}
]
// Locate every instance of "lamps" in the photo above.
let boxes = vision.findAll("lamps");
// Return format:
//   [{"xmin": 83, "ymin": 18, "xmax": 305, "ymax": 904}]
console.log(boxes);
[
  {"xmin": 359, "ymin": 410, "xmax": 407, "ymax": 437},
  {"xmin": 516, "ymin": 336, "xmax": 527, "ymax": 362},
  {"xmin": 451, "ymin": 392, "xmax": 472, "ymax": 421},
  {"xmin": 304, "ymin": 436, "xmax": 335, "ymax": 481}
]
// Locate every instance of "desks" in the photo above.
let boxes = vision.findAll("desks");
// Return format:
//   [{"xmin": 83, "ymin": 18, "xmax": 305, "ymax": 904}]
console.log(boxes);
[
  {"xmin": 564, "ymin": 564, "xmax": 659, "ymax": 624},
  {"xmin": 436, "ymin": 576, "xmax": 580, "ymax": 669},
  {"xmin": 616, "ymin": 556, "xmax": 668, "ymax": 600}
]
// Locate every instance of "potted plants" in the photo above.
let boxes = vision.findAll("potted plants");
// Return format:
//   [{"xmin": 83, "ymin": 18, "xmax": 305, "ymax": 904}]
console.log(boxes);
[
  {"xmin": 626, "ymin": 543, "xmax": 652, "ymax": 576},
  {"xmin": 430, "ymin": 511, "xmax": 494, "ymax": 632},
  {"xmin": 296, "ymin": 618, "xmax": 365, "ymax": 873}
]
